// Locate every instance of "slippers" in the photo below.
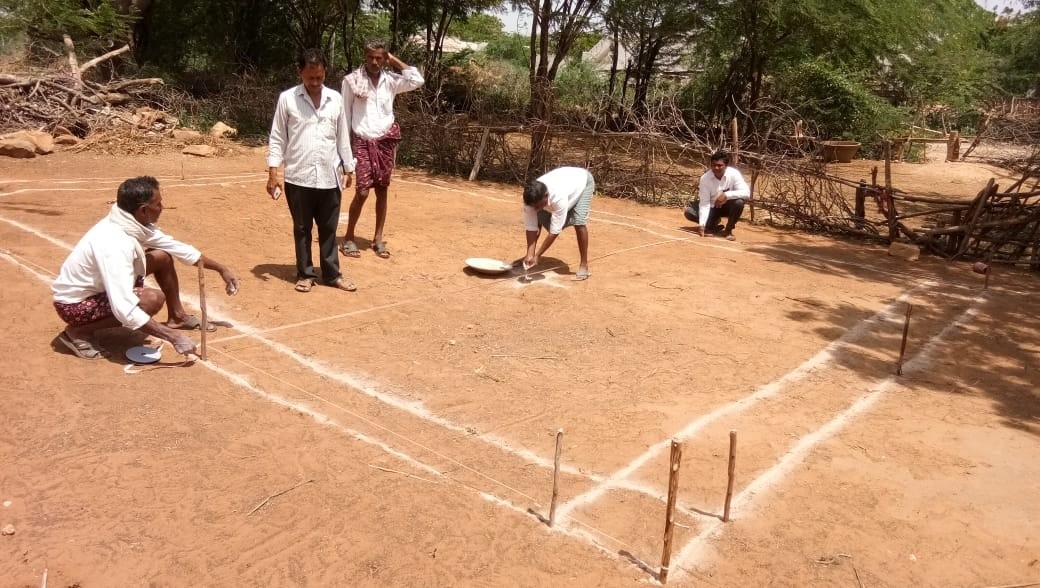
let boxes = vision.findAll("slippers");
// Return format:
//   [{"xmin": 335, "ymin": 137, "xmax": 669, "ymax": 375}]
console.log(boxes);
[
  {"xmin": 58, "ymin": 332, "xmax": 112, "ymax": 360},
  {"xmin": 177, "ymin": 314, "xmax": 218, "ymax": 333},
  {"xmin": 371, "ymin": 241, "xmax": 390, "ymax": 259},
  {"xmin": 572, "ymin": 273, "xmax": 591, "ymax": 280},
  {"xmin": 339, "ymin": 242, "xmax": 362, "ymax": 258}
]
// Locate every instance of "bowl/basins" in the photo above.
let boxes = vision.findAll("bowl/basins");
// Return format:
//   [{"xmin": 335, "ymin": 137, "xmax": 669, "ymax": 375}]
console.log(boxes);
[
  {"xmin": 465, "ymin": 258, "xmax": 514, "ymax": 274},
  {"xmin": 819, "ymin": 140, "xmax": 861, "ymax": 163},
  {"xmin": 779, "ymin": 135, "xmax": 815, "ymax": 154}
]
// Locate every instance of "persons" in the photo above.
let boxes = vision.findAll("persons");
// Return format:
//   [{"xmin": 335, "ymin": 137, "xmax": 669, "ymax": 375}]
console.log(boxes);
[
  {"xmin": 510, "ymin": 166, "xmax": 595, "ymax": 281},
  {"xmin": 50, "ymin": 175, "xmax": 239, "ymax": 360},
  {"xmin": 266, "ymin": 48, "xmax": 356, "ymax": 291},
  {"xmin": 341, "ymin": 39, "xmax": 424, "ymax": 258},
  {"xmin": 684, "ymin": 151, "xmax": 750, "ymax": 241}
]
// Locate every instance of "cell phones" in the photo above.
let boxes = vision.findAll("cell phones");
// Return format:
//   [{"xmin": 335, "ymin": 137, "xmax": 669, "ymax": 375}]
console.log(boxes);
[{"xmin": 273, "ymin": 185, "xmax": 282, "ymax": 200}]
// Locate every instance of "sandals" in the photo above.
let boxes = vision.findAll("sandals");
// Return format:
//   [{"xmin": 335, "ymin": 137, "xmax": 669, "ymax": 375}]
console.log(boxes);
[
  {"xmin": 325, "ymin": 276, "xmax": 358, "ymax": 290},
  {"xmin": 295, "ymin": 276, "xmax": 314, "ymax": 292}
]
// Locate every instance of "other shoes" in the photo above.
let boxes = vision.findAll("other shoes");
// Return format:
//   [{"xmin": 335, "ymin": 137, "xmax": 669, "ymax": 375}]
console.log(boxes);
[
  {"xmin": 723, "ymin": 230, "xmax": 735, "ymax": 240},
  {"xmin": 704, "ymin": 225, "xmax": 723, "ymax": 236}
]
